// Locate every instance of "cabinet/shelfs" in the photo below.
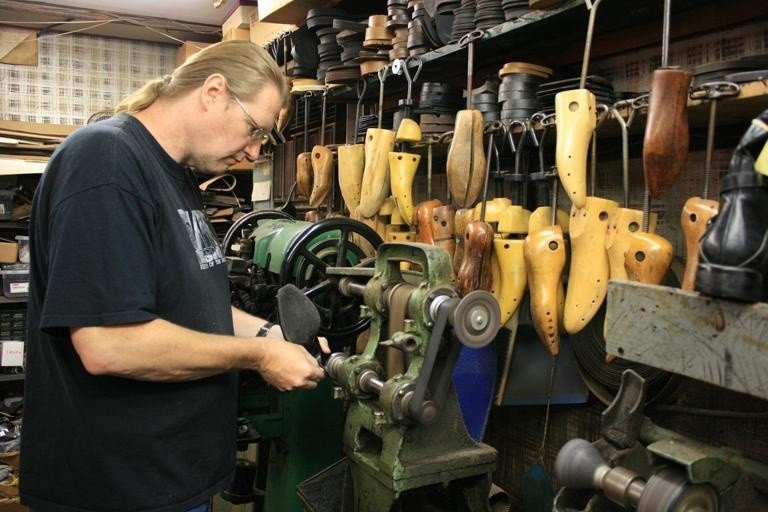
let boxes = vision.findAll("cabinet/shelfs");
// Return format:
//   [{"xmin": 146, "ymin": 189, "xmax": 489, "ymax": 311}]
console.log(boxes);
[
  {"xmin": 0, "ymin": 141, "xmax": 252, "ymax": 453},
  {"xmin": 320, "ymin": 2, "xmax": 767, "ymax": 170}
]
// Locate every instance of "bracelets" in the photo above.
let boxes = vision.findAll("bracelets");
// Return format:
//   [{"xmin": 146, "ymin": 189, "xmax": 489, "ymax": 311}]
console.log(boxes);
[{"xmin": 255, "ymin": 322, "xmax": 276, "ymax": 337}]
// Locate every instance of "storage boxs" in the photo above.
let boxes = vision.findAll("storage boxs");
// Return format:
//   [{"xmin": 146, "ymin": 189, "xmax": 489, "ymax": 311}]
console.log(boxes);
[
  {"xmin": 0, "ymin": 234, "xmax": 34, "ymax": 299},
  {"xmin": 1, "ymin": 338, "xmax": 26, "ymax": 368},
  {"xmin": 171, "ymin": 0, "xmax": 336, "ymax": 73}
]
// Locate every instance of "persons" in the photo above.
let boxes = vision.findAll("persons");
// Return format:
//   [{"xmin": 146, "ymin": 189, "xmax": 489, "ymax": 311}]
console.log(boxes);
[{"xmin": 18, "ymin": 40, "xmax": 331, "ymax": 512}]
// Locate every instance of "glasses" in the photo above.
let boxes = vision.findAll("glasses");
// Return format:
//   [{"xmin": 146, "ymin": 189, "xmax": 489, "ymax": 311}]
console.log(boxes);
[{"xmin": 226, "ymin": 79, "xmax": 272, "ymax": 146}]
